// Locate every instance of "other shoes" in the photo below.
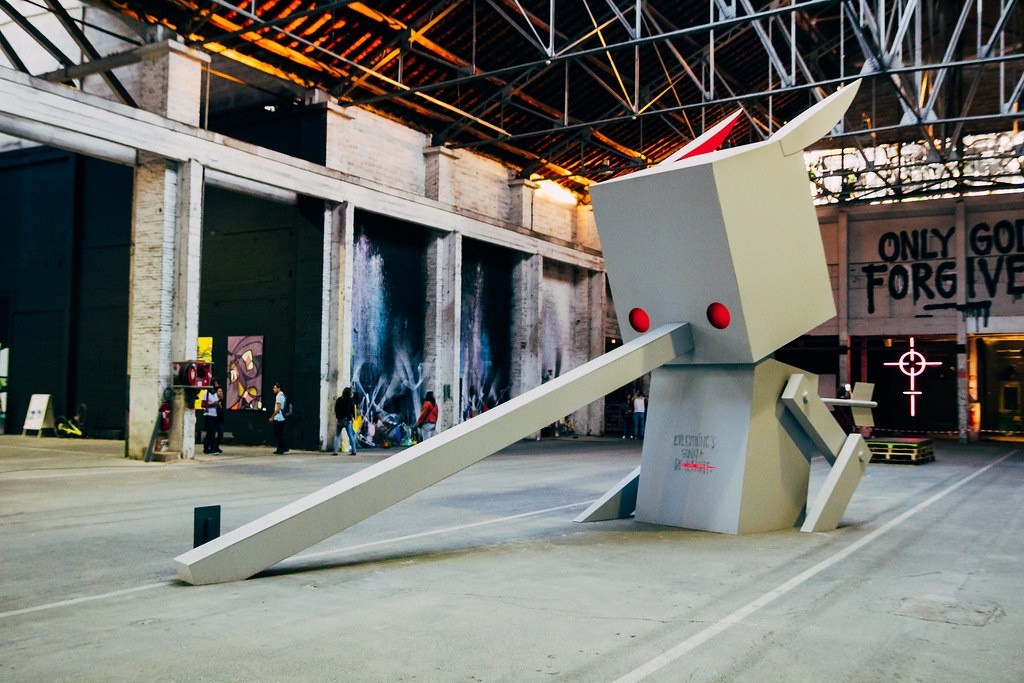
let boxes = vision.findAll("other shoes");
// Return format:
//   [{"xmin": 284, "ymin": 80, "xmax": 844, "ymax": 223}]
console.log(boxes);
[
  {"xmin": 203, "ymin": 448, "xmax": 223, "ymax": 454},
  {"xmin": 273, "ymin": 450, "xmax": 283, "ymax": 454},
  {"xmin": 351, "ymin": 453, "xmax": 355, "ymax": 455},
  {"xmin": 333, "ymin": 453, "xmax": 337, "ymax": 456}
]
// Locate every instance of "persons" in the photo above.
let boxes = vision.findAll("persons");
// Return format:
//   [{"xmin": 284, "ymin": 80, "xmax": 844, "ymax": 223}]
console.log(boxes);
[
  {"xmin": 268, "ymin": 382, "xmax": 289, "ymax": 455},
  {"xmin": 833, "ymin": 387, "xmax": 852, "ymax": 436},
  {"xmin": 622, "ymin": 388, "xmax": 647, "ymax": 440},
  {"xmin": 203, "ymin": 377, "xmax": 224, "ymax": 454},
  {"xmin": 330, "ymin": 387, "xmax": 358, "ymax": 456},
  {"xmin": 415, "ymin": 391, "xmax": 438, "ymax": 441}
]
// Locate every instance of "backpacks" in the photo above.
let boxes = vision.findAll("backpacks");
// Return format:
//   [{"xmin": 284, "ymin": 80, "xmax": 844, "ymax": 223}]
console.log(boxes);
[{"xmin": 278, "ymin": 394, "xmax": 293, "ymax": 417}]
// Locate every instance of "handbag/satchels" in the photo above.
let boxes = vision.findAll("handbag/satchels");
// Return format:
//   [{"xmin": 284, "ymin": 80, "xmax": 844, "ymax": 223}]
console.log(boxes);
[{"xmin": 418, "ymin": 422, "xmax": 423, "ymax": 428}]
